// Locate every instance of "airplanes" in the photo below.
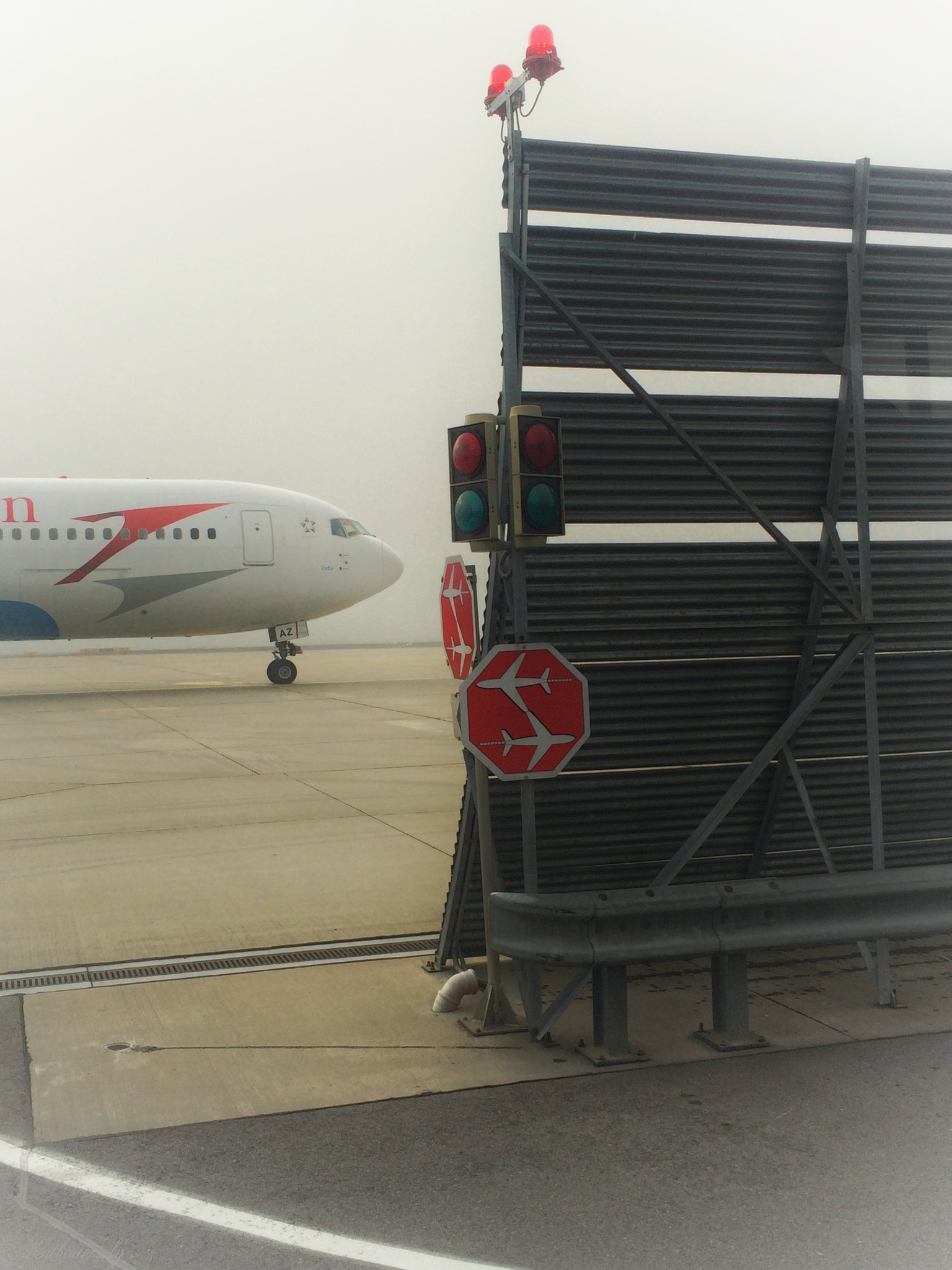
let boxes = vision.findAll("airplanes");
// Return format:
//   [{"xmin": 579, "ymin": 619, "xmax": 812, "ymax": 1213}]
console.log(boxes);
[{"xmin": 0, "ymin": 475, "xmax": 402, "ymax": 685}]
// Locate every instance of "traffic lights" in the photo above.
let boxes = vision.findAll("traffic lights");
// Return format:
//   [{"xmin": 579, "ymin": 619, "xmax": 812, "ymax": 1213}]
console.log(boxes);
[
  {"xmin": 503, "ymin": 404, "xmax": 566, "ymax": 549},
  {"xmin": 444, "ymin": 412, "xmax": 503, "ymax": 555}
]
